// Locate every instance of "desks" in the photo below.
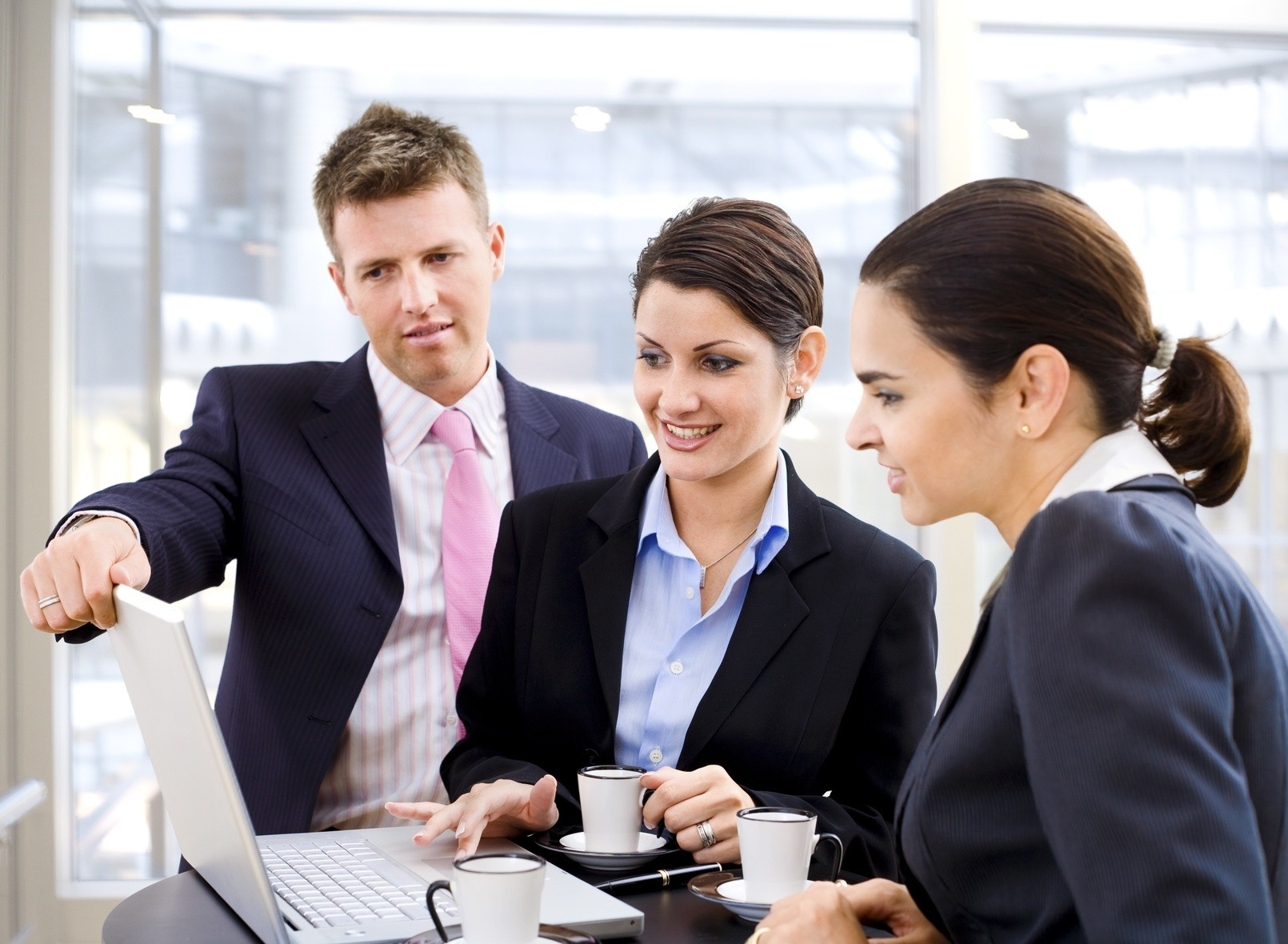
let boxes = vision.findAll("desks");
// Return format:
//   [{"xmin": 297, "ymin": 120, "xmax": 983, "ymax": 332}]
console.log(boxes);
[{"xmin": 101, "ymin": 825, "xmax": 899, "ymax": 944}]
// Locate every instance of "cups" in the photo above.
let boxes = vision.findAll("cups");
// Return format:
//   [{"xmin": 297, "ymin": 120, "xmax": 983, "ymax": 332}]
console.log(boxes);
[
  {"xmin": 735, "ymin": 807, "xmax": 842, "ymax": 902},
  {"xmin": 577, "ymin": 764, "xmax": 665, "ymax": 853},
  {"xmin": 427, "ymin": 853, "xmax": 545, "ymax": 944}
]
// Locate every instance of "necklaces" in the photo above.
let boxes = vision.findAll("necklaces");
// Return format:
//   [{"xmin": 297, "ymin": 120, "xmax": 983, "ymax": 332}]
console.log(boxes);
[{"xmin": 693, "ymin": 523, "xmax": 758, "ymax": 589}]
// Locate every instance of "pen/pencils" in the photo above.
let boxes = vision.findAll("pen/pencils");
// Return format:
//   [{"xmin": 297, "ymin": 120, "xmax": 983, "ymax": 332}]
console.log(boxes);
[{"xmin": 593, "ymin": 861, "xmax": 724, "ymax": 897}]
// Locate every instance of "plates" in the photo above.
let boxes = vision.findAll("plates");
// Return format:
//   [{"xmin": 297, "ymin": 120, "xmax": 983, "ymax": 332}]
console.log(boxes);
[
  {"xmin": 687, "ymin": 868, "xmax": 813, "ymax": 921},
  {"xmin": 531, "ymin": 822, "xmax": 683, "ymax": 871},
  {"xmin": 403, "ymin": 925, "xmax": 600, "ymax": 944}
]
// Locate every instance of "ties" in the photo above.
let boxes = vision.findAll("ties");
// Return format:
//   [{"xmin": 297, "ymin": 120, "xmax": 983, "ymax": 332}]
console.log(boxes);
[{"xmin": 429, "ymin": 411, "xmax": 504, "ymax": 741}]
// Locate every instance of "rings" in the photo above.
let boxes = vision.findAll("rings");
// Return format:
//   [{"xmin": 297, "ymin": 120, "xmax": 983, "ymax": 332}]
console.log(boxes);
[
  {"xmin": 37, "ymin": 593, "xmax": 62, "ymax": 612},
  {"xmin": 695, "ymin": 822, "xmax": 710, "ymax": 850},
  {"xmin": 746, "ymin": 926, "xmax": 769, "ymax": 943},
  {"xmin": 700, "ymin": 818, "xmax": 717, "ymax": 846}
]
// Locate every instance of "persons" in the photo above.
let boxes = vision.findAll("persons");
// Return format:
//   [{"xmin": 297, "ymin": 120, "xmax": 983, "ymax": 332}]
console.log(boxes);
[
  {"xmin": 15, "ymin": 98, "xmax": 654, "ymax": 880},
  {"xmin": 377, "ymin": 192, "xmax": 940, "ymax": 889},
  {"xmin": 734, "ymin": 175, "xmax": 1287, "ymax": 943}
]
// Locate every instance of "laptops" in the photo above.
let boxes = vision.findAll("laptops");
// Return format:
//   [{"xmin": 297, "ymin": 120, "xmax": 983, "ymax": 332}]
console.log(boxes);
[{"xmin": 109, "ymin": 582, "xmax": 645, "ymax": 944}]
